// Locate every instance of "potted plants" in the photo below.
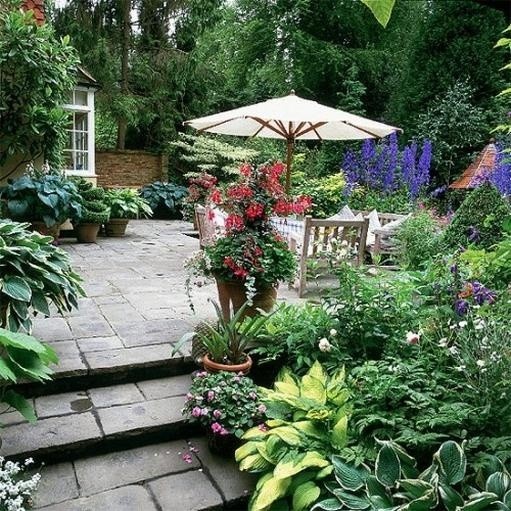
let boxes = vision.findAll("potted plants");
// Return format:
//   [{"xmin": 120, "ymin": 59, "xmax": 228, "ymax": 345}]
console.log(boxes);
[
  {"xmin": 2, "ymin": 174, "xmax": 154, "ymax": 245},
  {"xmin": 171, "ymin": 297, "xmax": 281, "ymax": 376}
]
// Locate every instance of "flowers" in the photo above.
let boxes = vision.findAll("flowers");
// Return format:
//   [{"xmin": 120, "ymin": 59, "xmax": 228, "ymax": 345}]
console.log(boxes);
[
  {"xmin": 189, "ymin": 163, "xmax": 312, "ymax": 307},
  {"xmin": 182, "ymin": 371, "xmax": 271, "ymax": 436},
  {"xmin": 0, "ymin": 455, "xmax": 45, "ymax": 510}
]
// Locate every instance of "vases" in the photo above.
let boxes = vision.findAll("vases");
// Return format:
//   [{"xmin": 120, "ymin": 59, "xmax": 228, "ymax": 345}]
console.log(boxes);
[
  {"xmin": 207, "ymin": 430, "xmax": 239, "ymax": 457},
  {"xmin": 212, "ymin": 272, "xmax": 276, "ymax": 324}
]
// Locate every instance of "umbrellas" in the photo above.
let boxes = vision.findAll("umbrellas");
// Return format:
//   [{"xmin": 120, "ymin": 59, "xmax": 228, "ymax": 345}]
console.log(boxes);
[{"xmin": 181, "ymin": 88, "xmax": 403, "ymax": 201}]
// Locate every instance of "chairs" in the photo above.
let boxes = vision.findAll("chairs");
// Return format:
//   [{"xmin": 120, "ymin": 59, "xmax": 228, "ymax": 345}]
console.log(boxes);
[
  {"xmin": 194, "ymin": 201, "xmax": 216, "ymax": 252},
  {"xmin": 287, "ymin": 215, "xmax": 371, "ymax": 298},
  {"xmin": 367, "ymin": 214, "xmax": 415, "ymax": 266}
]
